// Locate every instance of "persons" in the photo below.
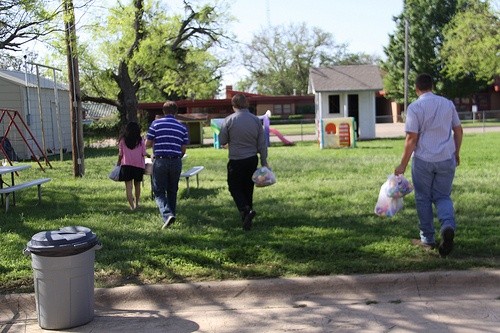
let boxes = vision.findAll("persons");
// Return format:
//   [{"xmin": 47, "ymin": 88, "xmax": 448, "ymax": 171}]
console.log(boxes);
[
  {"xmin": 116, "ymin": 122, "xmax": 152, "ymax": 209},
  {"xmin": 392, "ymin": 74, "xmax": 463, "ymax": 256},
  {"xmin": 217, "ymin": 92, "xmax": 270, "ymax": 230},
  {"xmin": 147, "ymin": 100, "xmax": 189, "ymax": 228}
]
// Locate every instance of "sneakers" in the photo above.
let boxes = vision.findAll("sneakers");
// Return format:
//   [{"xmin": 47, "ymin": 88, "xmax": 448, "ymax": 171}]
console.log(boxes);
[
  {"xmin": 242, "ymin": 209, "xmax": 256, "ymax": 230},
  {"xmin": 438, "ymin": 226, "xmax": 455, "ymax": 259},
  {"xmin": 162, "ymin": 216, "xmax": 175, "ymax": 229},
  {"xmin": 411, "ymin": 238, "xmax": 435, "ymax": 250}
]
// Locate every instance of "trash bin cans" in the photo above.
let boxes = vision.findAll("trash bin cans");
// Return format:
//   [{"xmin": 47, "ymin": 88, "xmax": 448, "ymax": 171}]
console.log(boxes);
[{"xmin": 27, "ymin": 225, "xmax": 98, "ymax": 329}]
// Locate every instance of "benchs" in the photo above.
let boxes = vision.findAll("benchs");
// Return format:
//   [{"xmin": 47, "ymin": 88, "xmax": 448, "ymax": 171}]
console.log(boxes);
[
  {"xmin": 0, "ymin": 177, "xmax": 51, "ymax": 213},
  {"xmin": 180, "ymin": 166, "xmax": 204, "ymax": 195}
]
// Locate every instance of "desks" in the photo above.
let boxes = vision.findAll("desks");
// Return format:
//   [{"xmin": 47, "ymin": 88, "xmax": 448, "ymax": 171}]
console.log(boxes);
[
  {"xmin": 145, "ymin": 154, "xmax": 189, "ymax": 201},
  {"xmin": 0, "ymin": 165, "xmax": 31, "ymax": 207}
]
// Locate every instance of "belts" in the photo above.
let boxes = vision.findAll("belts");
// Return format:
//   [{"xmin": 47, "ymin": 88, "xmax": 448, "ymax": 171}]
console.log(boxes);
[{"xmin": 156, "ymin": 156, "xmax": 181, "ymax": 159}]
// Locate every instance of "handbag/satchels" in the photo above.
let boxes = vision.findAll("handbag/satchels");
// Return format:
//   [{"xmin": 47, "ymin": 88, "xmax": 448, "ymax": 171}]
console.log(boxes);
[
  {"xmin": 252, "ymin": 167, "xmax": 275, "ymax": 187},
  {"xmin": 109, "ymin": 166, "xmax": 119, "ymax": 182},
  {"xmin": 374, "ymin": 174, "xmax": 414, "ymax": 216}
]
